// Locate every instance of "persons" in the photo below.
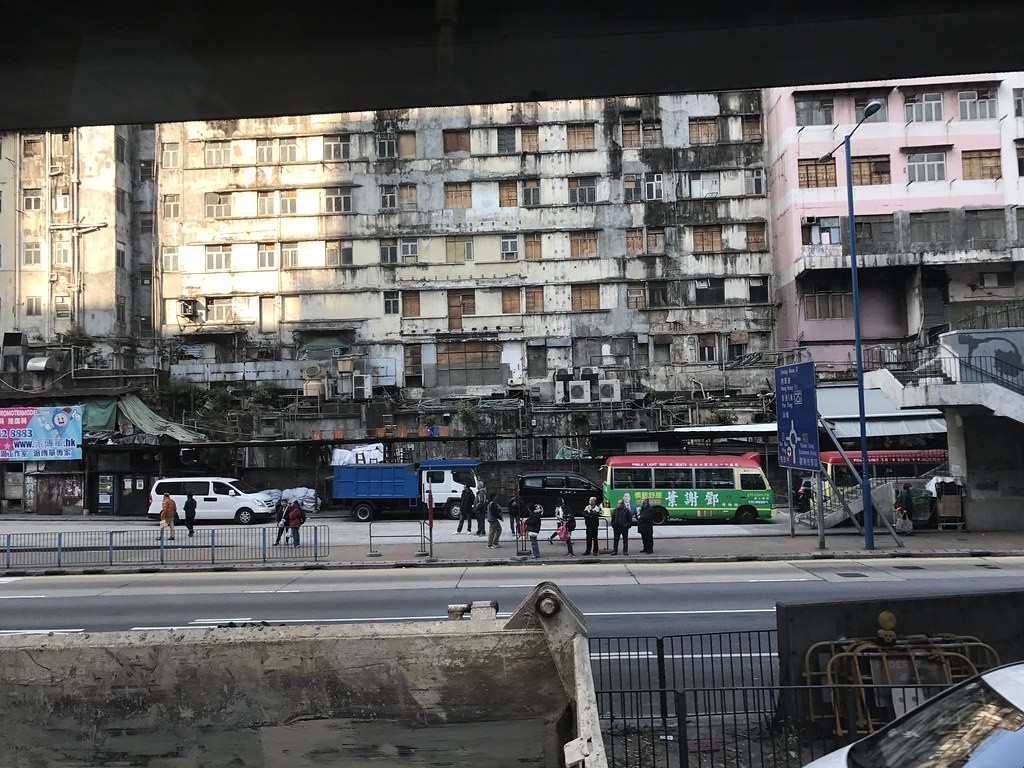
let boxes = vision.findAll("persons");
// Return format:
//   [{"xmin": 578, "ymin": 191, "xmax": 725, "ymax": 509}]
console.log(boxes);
[
  {"xmin": 893, "ymin": 483, "xmax": 916, "ymax": 528},
  {"xmin": 610, "ymin": 499, "xmax": 633, "ymax": 556},
  {"xmin": 798, "ymin": 480, "xmax": 813, "ymax": 514},
  {"xmin": 506, "ymin": 492, "xmax": 521, "ymax": 536},
  {"xmin": 271, "ymin": 499, "xmax": 293, "ymax": 547},
  {"xmin": 548, "ymin": 498, "xmax": 575, "ymax": 545},
  {"xmin": 288, "ymin": 501, "xmax": 303, "ymax": 549},
  {"xmin": 581, "ymin": 497, "xmax": 601, "ymax": 557},
  {"xmin": 636, "ymin": 497, "xmax": 654, "ymax": 554},
  {"xmin": 472, "ymin": 480, "xmax": 488, "ymax": 536},
  {"xmin": 183, "ymin": 492, "xmax": 197, "ymax": 538},
  {"xmin": 561, "ymin": 505, "xmax": 576, "ymax": 559},
  {"xmin": 622, "ymin": 492, "xmax": 637, "ymax": 517},
  {"xmin": 522, "ymin": 503, "xmax": 541, "ymax": 558},
  {"xmin": 485, "ymin": 492, "xmax": 504, "ymax": 549},
  {"xmin": 155, "ymin": 492, "xmax": 176, "ymax": 540},
  {"xmin": 453, "ymin": 483, "xmax": 475, "ymax": 535}
]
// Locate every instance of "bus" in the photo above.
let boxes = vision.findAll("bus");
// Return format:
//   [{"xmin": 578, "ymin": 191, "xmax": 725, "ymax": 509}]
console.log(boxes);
[
  {"xmin": 599, "ymin": 451, "xmax": 779, "ymax": 526},
  {"xmin": 810, "ymin": 449, "xmax": 949, "ymax": 527}
]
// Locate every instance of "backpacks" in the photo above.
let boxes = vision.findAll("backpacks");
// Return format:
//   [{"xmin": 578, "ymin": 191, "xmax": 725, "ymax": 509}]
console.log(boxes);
[
  {"xmin": 297, "ymin": 508, "xmax": 305, "ymax": 524},
  {"xmin": 567, "ymin": 516, "xmax": 576, "ymax": 531}
]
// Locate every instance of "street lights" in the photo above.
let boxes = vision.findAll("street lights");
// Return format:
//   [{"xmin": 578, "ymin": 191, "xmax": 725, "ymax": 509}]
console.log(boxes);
[{"xmin": 818, "ymin": 100, "xmax": 884, "ymax": 550}]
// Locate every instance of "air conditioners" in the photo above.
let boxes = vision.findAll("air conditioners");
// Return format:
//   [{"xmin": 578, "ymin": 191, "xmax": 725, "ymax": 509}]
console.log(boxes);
[
  {"xmin": 352, "ymin": 375, "xmax": 372, "ymax": 399},
  {"xmin": 555, "ymin": 367, "xmax": 573, "ymax": 375},
  {"xmin": 569, "ymin": 380, "xmax": 590, "ymax": 403},
  {"xmin": 599, "ymin": 379, "xmax": 621, "ymax": 402},
  {"xmin": 580, "ymin": 367, "xmax": 598, "ymax": 375}
]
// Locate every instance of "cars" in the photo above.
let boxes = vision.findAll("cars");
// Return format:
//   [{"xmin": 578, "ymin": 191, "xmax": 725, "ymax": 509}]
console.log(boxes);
[{"xmin": 799, "ymin": 660, "xmax": 1023, "ymax": 768}]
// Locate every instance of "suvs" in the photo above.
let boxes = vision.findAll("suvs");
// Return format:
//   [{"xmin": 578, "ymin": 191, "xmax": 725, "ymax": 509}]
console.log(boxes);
[{"xmin": 519, "ymin": 470, "xmax": 603, "ymax": 519}]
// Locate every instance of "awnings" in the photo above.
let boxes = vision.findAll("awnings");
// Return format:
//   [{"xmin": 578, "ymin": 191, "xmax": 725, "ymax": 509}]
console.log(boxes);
[{"xmin": 25, "ymin": 356, "xmax": 59, "ymax": 371}]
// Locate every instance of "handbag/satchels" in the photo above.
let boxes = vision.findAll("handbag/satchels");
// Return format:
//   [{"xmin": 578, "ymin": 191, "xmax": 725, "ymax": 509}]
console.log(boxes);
[
  {"xmin": 285, "ymin": 528, "xmax": 293, "ymax": 537},
  {"xmin": 895, "ymin": 512, "xmax": 914, "ymax": 534},
  {"xmin": 278, "ymin": 519, "xmax": 286, "ymax": 528},
  {"xmin": 160, "ymin": 519, "xmax": 167, "ymax": 529}
]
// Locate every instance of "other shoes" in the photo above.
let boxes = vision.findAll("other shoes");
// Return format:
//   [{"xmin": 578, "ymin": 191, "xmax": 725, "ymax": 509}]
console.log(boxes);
[
  {"xmin": 646, "ymin": 549, "xmax": 653, "ymax": 554},
  {"xmin": 640, "ymin": 549, "xmax": 648, "ymax": 552},
  {"xmin": 593, "ymin": 552, "xmax": 597, "ymax": 556},
  {"xmin": 623, "ymin": 551, "xmax": 629, "ymax": 555},
  {"xmin": 548, "ymin": 538, "xmax": 553, "ymax": 544},
  {"xmin": 155, "ymin": 536, "xmax": 161, "ymax": 539},
  {"xmin": 452, "ymin": 532, "xmax": 461, "ymax": 535},
  {"xmin": 611, "ymin": 551, "xmax": 617, "ymax": 554},
  {"xmin": 486, "ymin": 545, "xmax": 494, "ymax": 549},
  {"xmin": 582, "ymin": 552, "xmax": 590, "ymax": 555},
  {"xmin": 273, "ymin": 543, "xmax": 278, "ymax": 546},
  {"xmin": 466, "ymin": 531, "xmax": 472, "ymax": 535},
  {"xmin": 493, "ymin": 544, "xmax": 501, "ymax": 547},
  {"xmin": 188, "ymin": 530, "xmax": 194, "ymax": 537},
  {"xmin": 167, "ymin": 537, "xmax": 174, "ymax": 540},
  {"xmin": 564, "ymin": 552, "xmax": 575, "ymax": 558}
]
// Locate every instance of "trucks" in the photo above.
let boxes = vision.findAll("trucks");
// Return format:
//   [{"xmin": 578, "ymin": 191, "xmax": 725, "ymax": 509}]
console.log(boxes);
[{"xmin": 331, "ymin": 456, "xmax": 488, "ymax": 523}]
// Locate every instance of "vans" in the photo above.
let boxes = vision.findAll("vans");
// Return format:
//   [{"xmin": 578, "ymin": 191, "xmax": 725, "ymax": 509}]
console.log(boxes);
[{"xmin": 147, "ymin": 477, "xmax": 277, "ymax": 525}]
